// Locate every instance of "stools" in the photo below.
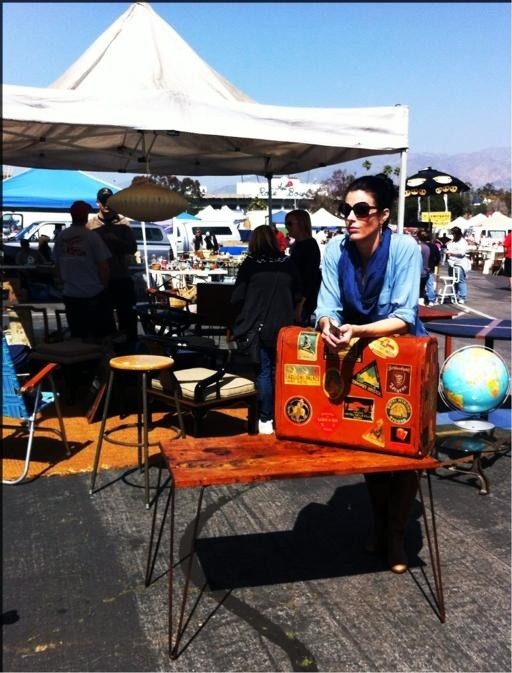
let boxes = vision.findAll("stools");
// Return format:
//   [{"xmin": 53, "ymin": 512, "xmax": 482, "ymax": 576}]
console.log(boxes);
[
  {"xmin": 141, "ymin": 367, "xmax": 260, "ymax": 434},
  {"xmin": 90, "ymin": 355, "xmax": 186, "ymax": 510}
]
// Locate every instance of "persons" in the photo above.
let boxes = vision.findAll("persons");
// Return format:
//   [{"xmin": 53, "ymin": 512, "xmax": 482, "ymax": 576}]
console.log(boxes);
[
  {"xmin": 193, "ymin": 229, "xmax": 203, "ymax": 251},
  {"xmin": 1, "ymin": 281, "xmax": 42, "ymax": 425},
  {"xmin": 86, "ymin": 188, "xmax": 138, "ymax": 347},
  {"xmin": 271, "ymin": 219, "xmax": 511, "ymax": 307},
  {"xmin": 0, "ymin": 234, "xmax": 55, "ymax": 289},
  {"xmin": 282, "ymin": 210, "xmax": 321, "ymax": 326},
  {"xmin": 314, "ymin": 175, "xmax": 423, "ymax": 576},
  {"xmin": 204, "ymin": 230, "xmax": 219, "ymax": 252},
  {"xmin": 52, "ymin": 200, "xmax": 114, "ymax": 342},
  {"xmin": 226, "ymin": 224, "xmax": 294, "ymax": 435}
]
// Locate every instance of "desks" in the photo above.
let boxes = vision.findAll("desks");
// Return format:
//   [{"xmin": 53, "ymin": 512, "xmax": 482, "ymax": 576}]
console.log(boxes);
[{"xmin": 146, "ymin": 434, "xmax": 446, "ymax": 659}]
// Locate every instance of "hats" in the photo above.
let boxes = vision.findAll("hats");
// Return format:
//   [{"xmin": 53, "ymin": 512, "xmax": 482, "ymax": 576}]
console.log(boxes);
[{"xmin": 98, "ymin": 188, "xmax": 112, "ymax": 198}]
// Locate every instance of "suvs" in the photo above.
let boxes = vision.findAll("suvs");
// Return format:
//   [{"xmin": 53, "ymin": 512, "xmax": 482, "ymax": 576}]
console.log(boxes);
[{"xmin": 1, "ymin": 218, "xmax": 172, "ymax": 300}]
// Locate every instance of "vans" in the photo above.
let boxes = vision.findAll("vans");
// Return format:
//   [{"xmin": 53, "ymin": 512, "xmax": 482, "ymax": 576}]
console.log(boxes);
[
  {"xmin": 160, "ymin": 218, "xmax": 242, "ymax": 252},
  {"xmin": 462, "ymin": 226, "xmax": 508, "ymax": 266}
]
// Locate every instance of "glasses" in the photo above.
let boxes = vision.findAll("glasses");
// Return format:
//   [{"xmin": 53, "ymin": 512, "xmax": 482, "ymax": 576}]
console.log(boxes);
[{"xmin": 339, "ymin": 202, "xmax": 375, "ymax": 216}]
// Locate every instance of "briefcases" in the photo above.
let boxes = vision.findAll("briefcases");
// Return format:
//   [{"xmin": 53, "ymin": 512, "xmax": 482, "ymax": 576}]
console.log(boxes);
[{"xmin": 274, "ymin": 326, "xmax": 438, "ymax": 458}]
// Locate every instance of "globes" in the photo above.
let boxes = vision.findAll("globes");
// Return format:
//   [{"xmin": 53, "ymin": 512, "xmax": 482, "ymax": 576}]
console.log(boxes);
[{"xmin": 438, "ymin": 344, "xmax": 511, "ymax": 432}]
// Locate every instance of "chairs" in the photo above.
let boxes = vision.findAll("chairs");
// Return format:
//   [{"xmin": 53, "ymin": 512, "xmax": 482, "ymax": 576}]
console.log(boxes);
[
  {"xmin": 2, "ymin": 333, "xmax": 71, "ymax": 485},
  {"xmin": 148, "ymin": 292, "xmax": 192, "ymax": 338},
  {"xmin": 55, "ymin": 309, "xmax": 68, "ymax": 338},
  {"xmin": 11, "ymin": 305, "xmax": 49, "ymax": 346}
]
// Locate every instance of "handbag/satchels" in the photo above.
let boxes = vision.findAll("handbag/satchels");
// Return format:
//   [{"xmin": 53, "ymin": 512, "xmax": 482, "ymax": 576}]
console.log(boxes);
[{"xmin": 238, "ymin": 334, "xmax": 260, "ymax": 364}]
